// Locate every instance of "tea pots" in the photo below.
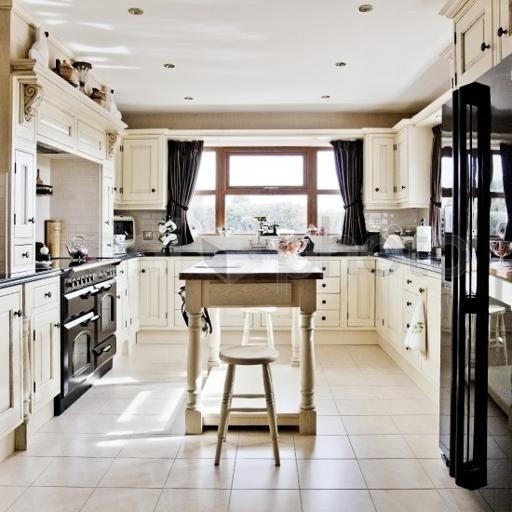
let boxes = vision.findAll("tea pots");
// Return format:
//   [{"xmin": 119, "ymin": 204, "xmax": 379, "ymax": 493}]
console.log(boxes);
[{"xmin": 66, "ymin": 236, "xmax": 89, "ymax": 259}]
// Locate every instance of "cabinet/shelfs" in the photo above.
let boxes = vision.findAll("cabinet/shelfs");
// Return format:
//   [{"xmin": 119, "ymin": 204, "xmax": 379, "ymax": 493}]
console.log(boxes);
[
  {"xmin": 116, "ymin": 257, "xmax": 212, "ymax": 356},
  {"xmin": 302, "ymin": 257, "xmax": 346, "ymax": 345},
  {"xmin": 342, "ymin": 256, "xmax": 378, "ymax": 345},
  {"xmin": 15, "ymin": 275, "xmax": 62, "ymax": 453},
  {"xmin": 219, "ymin": 305, "xmax": 292, "ymax": 345},
  {"xmin": 1, "ymin": 59, "xmax": 128, "ymax": 280},
  {"xmin": 361, "ymin": 119, "xmax": 432, "ymax": 212},
  {"xmin": 0, "ymin": 283, "xmax": 24, "ymax": 463},
  {"xmin": 114, "ymin": 127, "xmax": 169, "ymax": 211},
  {"xmin": 376, "ymin": 257, "xmax": 442, "ymax": 408}
]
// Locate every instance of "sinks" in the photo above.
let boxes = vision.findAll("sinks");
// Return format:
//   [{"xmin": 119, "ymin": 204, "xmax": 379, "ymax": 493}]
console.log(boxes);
[{"xmin": 216, "ymin": 250, "xmax": 280, "ymax": 254}]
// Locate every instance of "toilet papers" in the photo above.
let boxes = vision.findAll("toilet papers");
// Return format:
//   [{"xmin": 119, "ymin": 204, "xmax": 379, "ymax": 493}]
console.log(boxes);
[{"xmin": 415, "ymin": 225, "xmax": 431, "ymax": 252}]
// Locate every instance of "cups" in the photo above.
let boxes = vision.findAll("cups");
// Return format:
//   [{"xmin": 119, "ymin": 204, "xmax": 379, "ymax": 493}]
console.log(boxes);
[{"xmin": 158, "ymin": 218, "xmax": 178, "ymax": 246}]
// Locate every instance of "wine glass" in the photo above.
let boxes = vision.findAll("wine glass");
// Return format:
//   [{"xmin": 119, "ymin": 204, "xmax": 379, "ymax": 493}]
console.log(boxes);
[{"xmin": 490, "ymin": 241, "xmax": 512, "ymax": 266}]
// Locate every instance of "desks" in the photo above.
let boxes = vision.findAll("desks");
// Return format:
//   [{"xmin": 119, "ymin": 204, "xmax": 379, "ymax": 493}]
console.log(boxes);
[
  {"xmin": 489, "ymin": 274, "xmax": 512, "ymax": 431},
  {"xmin": 178, "ymin": 254, "xmax": 324, "ymax": 435}
]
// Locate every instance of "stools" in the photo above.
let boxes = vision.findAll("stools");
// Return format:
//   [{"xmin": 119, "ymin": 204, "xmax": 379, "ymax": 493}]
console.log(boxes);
[
  {"xmin": 488, "ymin": 304, "xmax": 508, "ymax": 366},
  {"xmin": 213, "ymin": 347, "xmax": 280, "ymax": 467},
  {"xmin": 239, "ymin": 307, "xmax": 277, "ymax": 347}
]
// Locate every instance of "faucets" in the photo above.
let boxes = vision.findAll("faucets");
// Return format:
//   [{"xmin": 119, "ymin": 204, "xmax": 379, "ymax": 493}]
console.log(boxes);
[{"xmin": 256, "ymin": 217, "xmax": 268, "ymax": 244}]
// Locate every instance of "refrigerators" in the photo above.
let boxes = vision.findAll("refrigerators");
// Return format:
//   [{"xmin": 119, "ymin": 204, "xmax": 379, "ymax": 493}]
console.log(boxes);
[{"xmin": 437, "ymin": 53, "xmax": 510, "ymax": 511}]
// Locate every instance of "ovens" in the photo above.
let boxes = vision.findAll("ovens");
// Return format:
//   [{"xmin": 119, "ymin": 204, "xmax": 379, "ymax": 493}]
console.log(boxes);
[{"xmin": 53, "ymin": 258, "xmax": 122, "ymax": 417}]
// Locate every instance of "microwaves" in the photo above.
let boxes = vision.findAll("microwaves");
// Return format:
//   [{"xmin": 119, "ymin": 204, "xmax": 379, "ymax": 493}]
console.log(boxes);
[{"xmin": 113, "ymin": 216, "xmax": 136, "ymax": 248}]
